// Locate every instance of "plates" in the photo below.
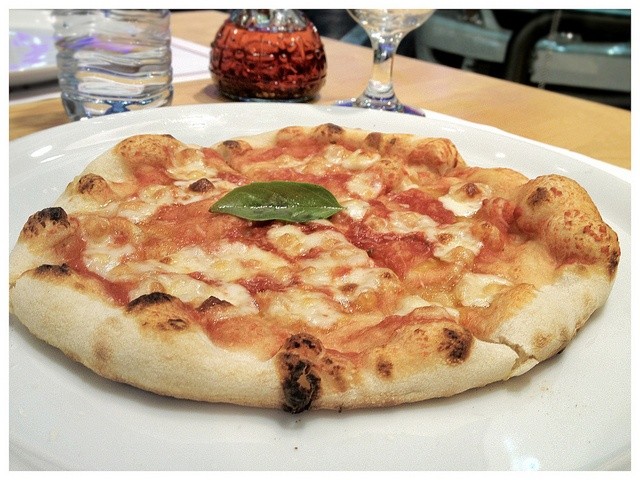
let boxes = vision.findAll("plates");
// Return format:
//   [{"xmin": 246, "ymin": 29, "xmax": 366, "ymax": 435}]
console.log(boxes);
[
  {"xmin": 9, "ymin": 102, "xmax": 631, "ymax": 471},
  {"xmin": 9, "ymin": 23, "xmax": 60, "ymax": 86}
]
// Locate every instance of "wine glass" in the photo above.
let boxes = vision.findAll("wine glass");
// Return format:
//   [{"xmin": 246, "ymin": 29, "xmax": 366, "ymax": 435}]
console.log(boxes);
[{"xmin": 333, "ymin": 9, "xmax": 437, "ymax": 121}]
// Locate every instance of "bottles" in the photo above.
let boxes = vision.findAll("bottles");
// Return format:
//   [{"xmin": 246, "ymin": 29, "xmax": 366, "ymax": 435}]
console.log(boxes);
[{"xmin": 49, "ymin": 10, "xmax": 176, "ymax": 120}]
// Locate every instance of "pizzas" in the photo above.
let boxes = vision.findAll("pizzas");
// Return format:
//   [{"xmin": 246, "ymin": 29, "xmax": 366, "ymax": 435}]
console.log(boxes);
[{"xmin": 10, "ymin": 123, "xmax": 620, "ymax": 411}]
